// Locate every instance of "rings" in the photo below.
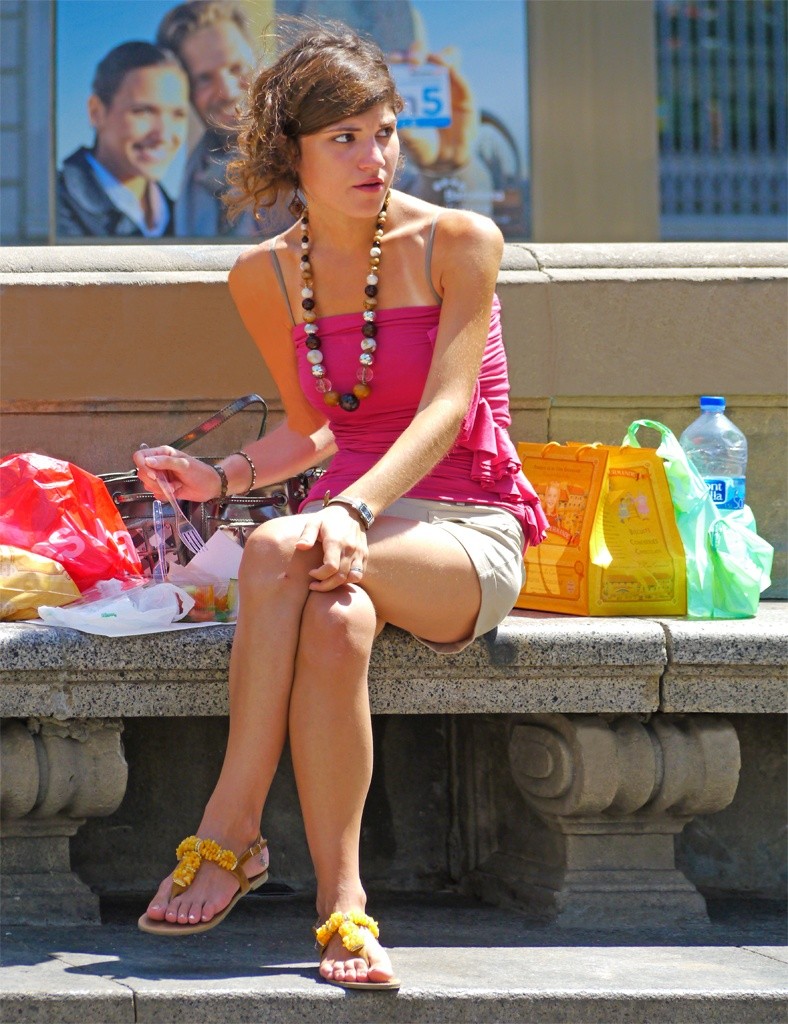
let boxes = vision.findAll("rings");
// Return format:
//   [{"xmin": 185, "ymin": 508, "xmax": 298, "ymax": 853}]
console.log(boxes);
[{"xmin": 351, "ymin": 568, "xmax": 363, "ymax": 573}]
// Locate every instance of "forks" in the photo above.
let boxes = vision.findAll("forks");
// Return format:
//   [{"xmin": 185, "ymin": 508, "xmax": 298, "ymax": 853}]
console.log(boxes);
[{"xmin": 139, "ymin": 442, "xmax": 210, "ymax": 556}]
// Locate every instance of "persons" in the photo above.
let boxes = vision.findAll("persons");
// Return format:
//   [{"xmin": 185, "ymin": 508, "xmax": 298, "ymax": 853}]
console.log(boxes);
[
  {"xmin": 56, "ymin": 42, "xmax": 190, "ymax": 238},
  {"xmin": 156, "ymin": 0, "xmax": 472, "ymax": 236},
  {"xmin": 132, "ymin": 28, "xmax": 549, "ymax": 989}
]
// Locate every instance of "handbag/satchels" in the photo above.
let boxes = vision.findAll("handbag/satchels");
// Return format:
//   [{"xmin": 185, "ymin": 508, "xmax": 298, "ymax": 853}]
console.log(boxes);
[
  {"xmin": 96, "ymin": 394, "xmax": 324, "ymax": 582},
  {"xmin": 0, "ymin": 453, "xmax": 144, "ymax": 602},
  {"xmin": 0, "ymin": 543, "xmax": 82, "ymax": 620},
  {"xmin": 622, "ymin": 419, "xmax": 775, "ymax": 620},
  {"xmin": 508, "ymin": 440, "xmax": 684, "ymax": 616}
]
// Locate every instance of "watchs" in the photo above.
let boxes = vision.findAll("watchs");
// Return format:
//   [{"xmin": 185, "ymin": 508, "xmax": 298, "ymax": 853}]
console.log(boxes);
[{"xmin": 322, "ymin": 490, "xmax": 374, "ymax": 530}]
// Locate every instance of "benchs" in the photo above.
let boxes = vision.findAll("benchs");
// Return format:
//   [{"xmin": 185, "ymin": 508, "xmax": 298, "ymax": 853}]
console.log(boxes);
[{"xmin": 0, "ymin": 601, "xmax": 788, "ymax": 926}]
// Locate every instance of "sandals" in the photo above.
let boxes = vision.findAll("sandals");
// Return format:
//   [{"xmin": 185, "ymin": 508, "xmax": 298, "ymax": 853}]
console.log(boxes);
[
  {"xmin": 313, "ymin": 911, "xmax": 400, "ymax": 989},
  {"xmin": 139, "ymin": 832, "xmax": 269, "ymax": 937}
]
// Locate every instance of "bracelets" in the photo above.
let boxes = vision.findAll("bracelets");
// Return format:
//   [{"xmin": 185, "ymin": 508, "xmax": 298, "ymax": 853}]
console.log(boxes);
[
  {"xmin": 234, "ymin": 451, "xmax": 256, "ymax": 495},
  {"xmin": 207, "ymin": 464, "xmax": 228, "ymax": 503}
]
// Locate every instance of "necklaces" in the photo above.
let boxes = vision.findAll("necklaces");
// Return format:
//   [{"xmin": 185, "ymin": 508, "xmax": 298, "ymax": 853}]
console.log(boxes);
[{"xmin": 300, "ymin": 190, "xmax": 390, "ymax": 411}]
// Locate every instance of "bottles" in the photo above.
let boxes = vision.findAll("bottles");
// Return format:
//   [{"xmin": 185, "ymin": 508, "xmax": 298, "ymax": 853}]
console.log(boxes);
[{"xmin": 680, "ymin": 395, "xmax": 747, "ymax": 527}]
